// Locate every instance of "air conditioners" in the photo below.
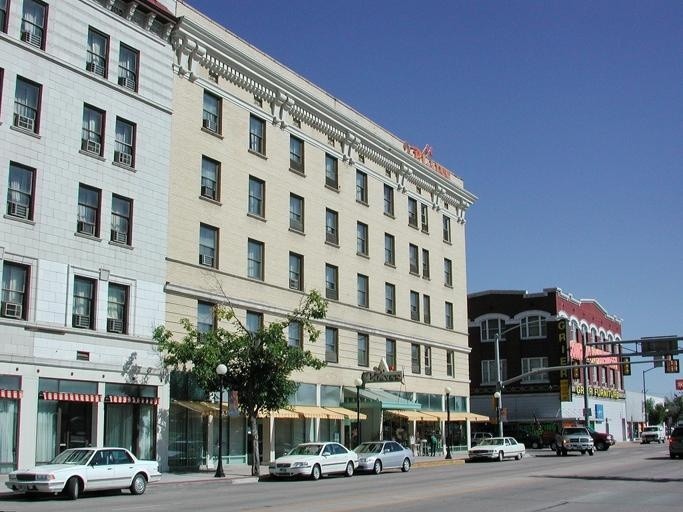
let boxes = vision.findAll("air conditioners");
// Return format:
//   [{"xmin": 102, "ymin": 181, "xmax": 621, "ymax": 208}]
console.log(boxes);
[
  {"xmin": 202, "ymin": 186, "xmax": 214, "ymax": 199},
  {"xmin": 199, "ymin": 254, "xmax": 213, "ymax": 266},
  {"xmin": 289, "ymin": 278, "xmax": 298, "ymax": 289},
  {"xmin": 205, "ymin": 119, "xmax": 216, "ymax": 129}
]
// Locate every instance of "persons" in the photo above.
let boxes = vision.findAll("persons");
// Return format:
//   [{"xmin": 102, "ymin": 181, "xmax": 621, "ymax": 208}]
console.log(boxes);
[{"xmin": 381, "ymin": 425, "xmax": 436, "ymax": 457}]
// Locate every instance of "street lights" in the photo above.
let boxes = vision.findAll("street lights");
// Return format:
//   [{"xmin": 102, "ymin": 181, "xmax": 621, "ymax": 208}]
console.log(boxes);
[
  {"xmin": 493, "ymin": 390, "xmax": 503, "ymax": 437},
  {"xmin": 354, "ymin": 376, "xmax": 361, "ymax": 448},
  {"xmin": 642, "ymin": 365, "xmax": 660, "ymax": 426},
  {"xmin": 493, "ymin": 314, "xmax": 567, "ymax": 438},
  {"xmin": 216, "ymin": 362, "xmax": 228, "ymax": 479},
  {"xmin": 443, "ymin": 386, "xmax": 454, "ymax": 459}
]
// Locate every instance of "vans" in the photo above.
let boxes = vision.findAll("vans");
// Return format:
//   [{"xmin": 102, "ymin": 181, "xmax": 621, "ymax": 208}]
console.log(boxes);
[{"xmin": 640, "ymin": 422, "xmax": 665, "ymax": 444}]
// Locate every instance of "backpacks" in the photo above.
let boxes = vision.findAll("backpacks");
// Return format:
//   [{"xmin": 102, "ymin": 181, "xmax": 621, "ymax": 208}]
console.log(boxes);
[{"xmin": 431, "ymin": 435, "xmax": 437, "ymax": 444}]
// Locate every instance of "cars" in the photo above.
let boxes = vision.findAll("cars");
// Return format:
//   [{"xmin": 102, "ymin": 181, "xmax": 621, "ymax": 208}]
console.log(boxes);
[
  {"xmin": 504, "ymin": 426, "xmax": 615, "ymax": 455},
  {"xmin": 665, "ymin": 424, "xmax": 683, "ymax": 458},
  {"xmin": 268, "ymin": 436, "xmax": 358, "ymax": 480},
  {"xmin": 471, "ymin": 431, "xmax": 495, "ymax": 447},
  {"xmin": 6, "ymin": 447, "xmax": 161, "ymax": 501},
  {"xmin": 468, "ymin": 437, "xmax": 527, "ymax": 460},
  {"xmin": 350, "ymin": 438, "xmax": 414, "ymax": 475}
]
